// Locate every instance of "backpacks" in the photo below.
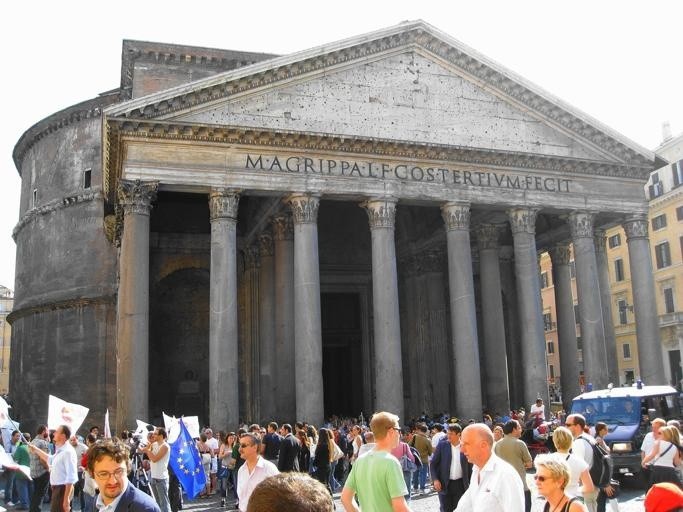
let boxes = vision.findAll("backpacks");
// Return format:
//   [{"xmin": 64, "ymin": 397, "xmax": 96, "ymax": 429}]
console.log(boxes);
[{"xmin": 576, "ymin": 436, "xmax": 613, "ymax": 487}]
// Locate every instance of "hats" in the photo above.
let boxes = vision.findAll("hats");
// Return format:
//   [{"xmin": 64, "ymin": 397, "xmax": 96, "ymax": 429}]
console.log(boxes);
[{"xmin": 644, "ymin": 482, "xmax": 683, "ymax": 512}]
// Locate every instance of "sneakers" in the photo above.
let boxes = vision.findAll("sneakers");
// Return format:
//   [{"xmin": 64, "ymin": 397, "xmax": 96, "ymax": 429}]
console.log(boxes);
[{"xmin": 0, "ymin": 501, "xmax": 15, "ymax": 512}]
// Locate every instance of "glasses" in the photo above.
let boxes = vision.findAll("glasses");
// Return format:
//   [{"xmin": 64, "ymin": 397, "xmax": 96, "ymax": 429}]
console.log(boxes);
[
  {"xmin": 533, "ymin": 475, "xmax": 554, "ymax": 482},
  {"xmin": 389, "ymin": 426, "xmax": 402, "ymax": 439},
  {"xmin": 239, "ymin": 442, "xmax": 252, "ymax": 448},
  {"xmin": 93, "ymin": 465, "xmax": 127, "ymax": 481}
]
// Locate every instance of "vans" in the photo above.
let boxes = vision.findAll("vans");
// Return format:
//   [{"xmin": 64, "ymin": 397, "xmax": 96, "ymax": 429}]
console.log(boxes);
[{"xmin": 570, "ymin": 381, "xmax": 679, "ymax": 487}]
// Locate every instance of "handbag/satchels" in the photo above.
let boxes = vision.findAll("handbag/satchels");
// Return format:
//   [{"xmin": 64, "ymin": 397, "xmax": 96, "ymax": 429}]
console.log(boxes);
[
  {"xmin": 202, "ymin": 453, "xmax": 212, "ymax": 465},
  {"xmin": 638, "ymin": 463, "xmax": 655, "ymax": 486},
  {"xmin": 333, "ymin": 444, "xmax": 344, "ymax": 461},
  {"xmin": 216, "ymin": 468, "xmax": 229, "ymax": 478},
  {"xmin": 400, "ymin": 454, "xmax": 418, "ymax": 472}
]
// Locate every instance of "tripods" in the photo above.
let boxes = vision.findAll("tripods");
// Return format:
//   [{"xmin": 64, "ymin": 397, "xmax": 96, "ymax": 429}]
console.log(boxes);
[{"xmin": 130, "ymin": 454, "xmax": 157, "ymax": 502}]
